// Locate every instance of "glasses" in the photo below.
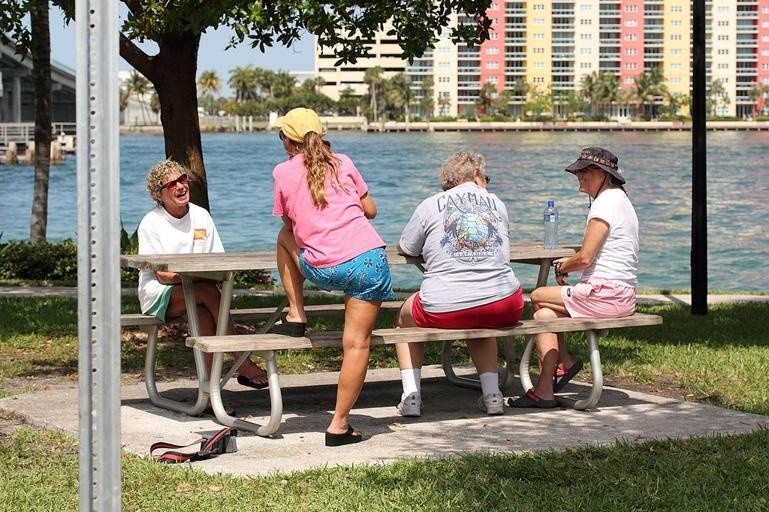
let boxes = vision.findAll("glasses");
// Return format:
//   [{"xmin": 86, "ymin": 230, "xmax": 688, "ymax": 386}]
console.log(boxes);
[
  {"xmin": 279, "ymin": 131, "xmax": 285, "ymax": 140},
  {"xmin": 157, "ymin": 173, "xmax": 187, "ymax": 189}
]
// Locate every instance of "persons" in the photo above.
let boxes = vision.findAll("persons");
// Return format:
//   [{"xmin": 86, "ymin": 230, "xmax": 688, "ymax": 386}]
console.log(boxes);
[
  {"xmin": 270, "ymin": 106, "xmax": 396, "ymax": 447},
  {"xmin": 397, "ymin": 149, "xmax": 526, "ymax": 418},
  {"xmin": 136, "ymin": 156, "xmax": 269, "ymax": 417},
  {"xmin": 506, "ymin": 147, "xmax": 641, "ymax": 409}
]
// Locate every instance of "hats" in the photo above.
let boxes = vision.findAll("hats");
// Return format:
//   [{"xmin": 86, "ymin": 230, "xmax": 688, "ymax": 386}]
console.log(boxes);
[
  {"xmin": 276, "ymin": 107, "xmax": 323, "ymax": 143},
  {"xmin": 565, "ymin": 147, "xmax": 625, "ymax": 185}
]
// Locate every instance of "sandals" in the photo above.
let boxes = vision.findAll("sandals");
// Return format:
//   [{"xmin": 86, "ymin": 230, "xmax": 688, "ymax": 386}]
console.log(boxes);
[
  {"xmin": 237, "ymin": 363, "xmax": 268, "ymax": 389},
  {"xmin": 203, "ymin": 404, "xmax": 236, "ymax": 416}
]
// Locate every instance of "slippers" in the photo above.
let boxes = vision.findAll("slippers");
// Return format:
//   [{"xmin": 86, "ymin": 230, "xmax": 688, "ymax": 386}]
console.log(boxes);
[
  {"xmin": 270, "ymin": 316, "xmax": 306, "ymax": 337},
  {"xmin": 326, "ymin": 424, "xmax": 361, "ymax": 446},
  {"xmin": 508, "ymin": 389, "xmax": 557, "ymax": 408},
  {"xmin": 553, "ymin": 360, "xmax": 583, "ymax": 393}
]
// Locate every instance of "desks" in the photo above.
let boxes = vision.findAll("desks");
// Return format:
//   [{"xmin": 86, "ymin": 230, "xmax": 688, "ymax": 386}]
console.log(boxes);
[{"xmin": 120, "ymin": 238, "xmax": 584, "ymax": 435}]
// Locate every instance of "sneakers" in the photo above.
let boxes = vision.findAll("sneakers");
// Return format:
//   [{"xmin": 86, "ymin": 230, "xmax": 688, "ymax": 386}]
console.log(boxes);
[
  {"xmin": 477, "ymin": 392, "xmax": 504, "ymax": 414},
  {"xmin": 397, "ymin": 392, "xmax": 424, "ymax": 417}
]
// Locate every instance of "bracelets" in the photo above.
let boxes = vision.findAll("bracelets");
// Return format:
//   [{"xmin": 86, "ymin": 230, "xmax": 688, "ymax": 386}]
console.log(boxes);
[{"xmin": 555, "ymin": 261, "xmax": 568, "ymax": 277}]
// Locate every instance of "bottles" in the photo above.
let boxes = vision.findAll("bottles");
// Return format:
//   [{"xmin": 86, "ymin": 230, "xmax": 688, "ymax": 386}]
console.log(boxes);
[{"xmin": 543, "ymin": 201, "xmax": 560, "ymax": 249}]
